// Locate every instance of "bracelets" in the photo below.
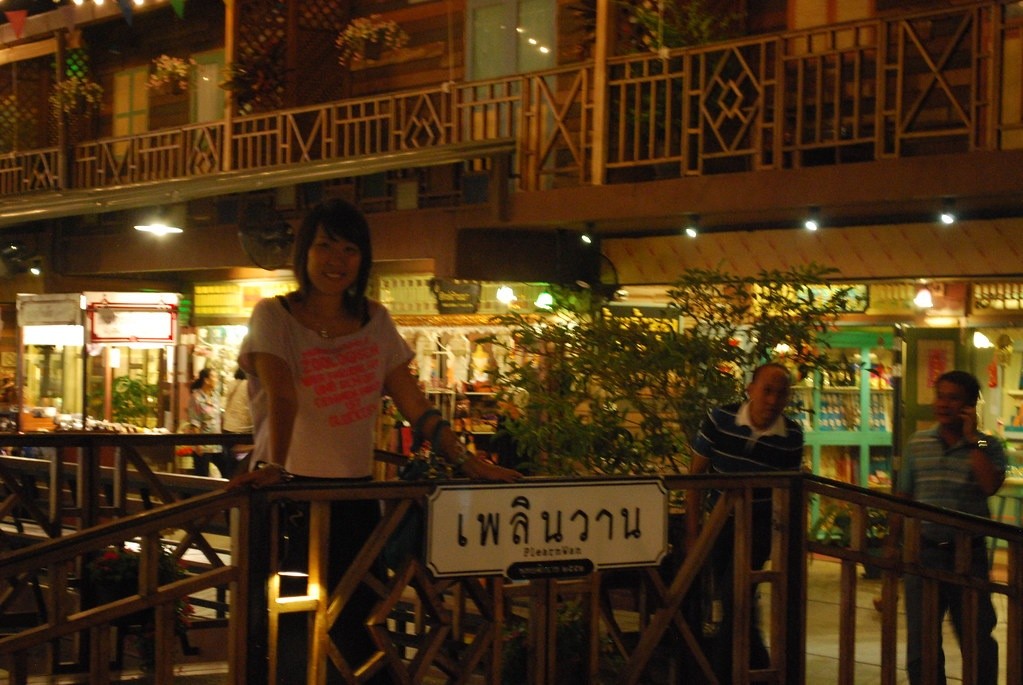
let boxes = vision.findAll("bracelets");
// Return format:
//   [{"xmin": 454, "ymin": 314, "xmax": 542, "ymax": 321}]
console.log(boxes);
[
  {"xmin": 413, "ymin": 409, "xmax": 450, "ymax": 452},
  {"xmin": 455, "ymin": 451, "xmax": 474, "ymax": 470},
  {"xmin": 944, "ymin": 436, "xmax": 992, "ymax": 458}
]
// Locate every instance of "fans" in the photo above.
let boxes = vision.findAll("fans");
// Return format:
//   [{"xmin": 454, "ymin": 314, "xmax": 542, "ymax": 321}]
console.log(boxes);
[{"xmin": 238, "ymin": 202, "xmax": 295, "ymax": 270}]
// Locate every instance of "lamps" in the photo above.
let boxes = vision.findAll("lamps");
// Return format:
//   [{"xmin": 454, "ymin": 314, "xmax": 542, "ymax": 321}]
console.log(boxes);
[{"xmin": 427, "ymin": 276, "xmax": 482, "ymax": 315}]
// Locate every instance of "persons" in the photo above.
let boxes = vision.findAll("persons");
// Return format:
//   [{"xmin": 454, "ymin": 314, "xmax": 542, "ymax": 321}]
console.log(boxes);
[
  {"xmin": 874, "ymin": 370, "xmax": 1007, "ymax": 685},
  {"xmin": 0, "ymin": 378, "xmax": 17, "ymax": 405},
  {"xmin": 684, "ymin": 362, "xmax": 804, "ymax": 671},
  {"xmin": 184, "ymin": 367, "xmax": 231, "ymax": 478},
  {"xmin": 222, "ymin": 367, "xmax": 255, "ymax": 436},
  {"xmin": 233, "ymin": 198, "xmax": 526, "ymax": 685}
]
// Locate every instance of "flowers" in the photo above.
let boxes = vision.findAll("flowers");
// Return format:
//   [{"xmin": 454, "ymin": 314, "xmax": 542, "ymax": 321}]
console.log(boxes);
[
  {"xmin": 62, "ymin": 544, "xmax": 195, "ymax": 629},
  {"xmin": 334, "ymin": 14, "xmax": 409, "ymax": 67},
  {"xmin": 145, "ymin": 54, "xmax": 192, "ymax": 93},
  {"xmin": 49, "ymin": 77, "xmax": 107, "ymax": 118}
]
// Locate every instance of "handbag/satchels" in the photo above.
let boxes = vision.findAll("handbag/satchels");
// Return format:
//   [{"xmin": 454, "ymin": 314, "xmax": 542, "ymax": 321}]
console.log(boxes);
[{"xmin": 382, "ymin": 409, "xmax": 451, "ymax": 587}]
